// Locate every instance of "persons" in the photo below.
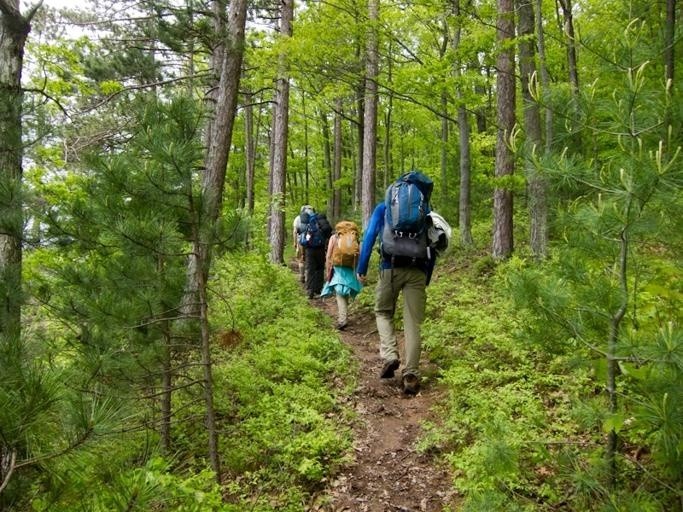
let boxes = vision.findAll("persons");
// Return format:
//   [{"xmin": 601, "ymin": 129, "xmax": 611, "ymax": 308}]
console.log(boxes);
[
  {"xmin": 293, "ymin": 205, "xmax": 332, "ymax": 299},
  {"xmin": 357, "ymin": 171, "xmax": 453, "ymax": 394},
  {"xmin": 319, "ymin": 219, "xmax": 364, "ymax": 330}
]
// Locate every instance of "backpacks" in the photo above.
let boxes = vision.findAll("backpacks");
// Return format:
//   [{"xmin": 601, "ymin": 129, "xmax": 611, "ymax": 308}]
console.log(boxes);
[
  {"xmin": 299, "ymin": 204, "xmax": 331, "ymax": 246},
  {"xmin": 382, "ymin": 172, "xmax": 429, "ymax": 259},
  {"xmin": 333, "ymin": 220, "xmax": 358, "ymax": 268}
]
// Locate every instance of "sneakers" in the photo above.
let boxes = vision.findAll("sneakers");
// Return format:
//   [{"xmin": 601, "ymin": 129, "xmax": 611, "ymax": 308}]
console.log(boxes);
[
  {"xmin": 299, "ymin": 277, "xmax": 321, "ymax": 300},
  {"xmin": 401, "ymin": 372, "xmax": 420, "ymax": 394},
  {"xmin": 336, "ymin": 320, "xmax": 349, "ymax": 332},
  {"xmin": 380, "ymin": 358, "xmax": 400, "ymax": 380}
]
